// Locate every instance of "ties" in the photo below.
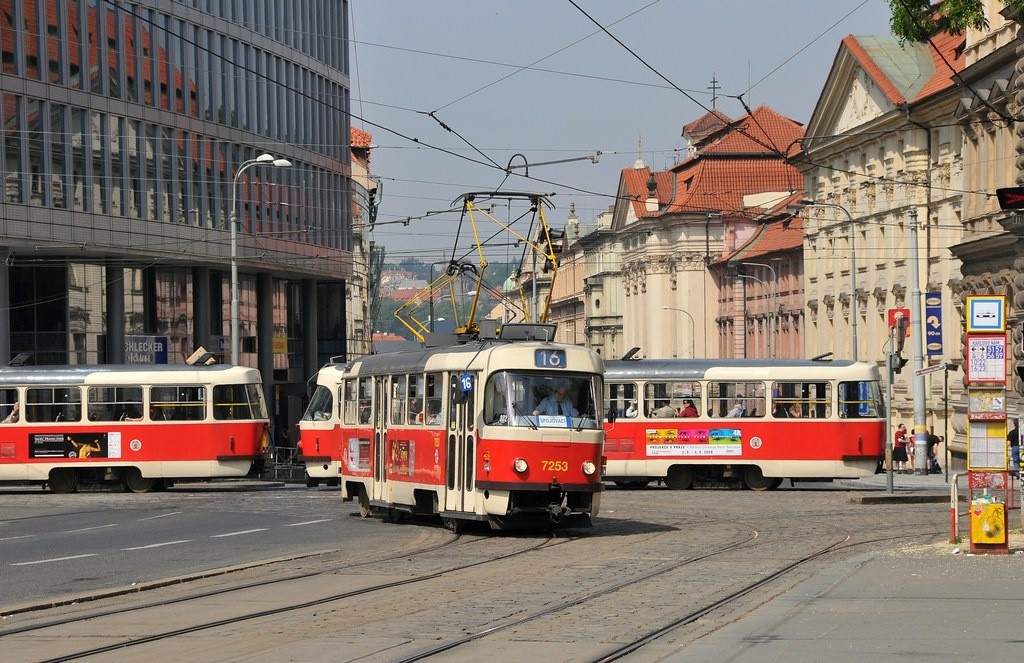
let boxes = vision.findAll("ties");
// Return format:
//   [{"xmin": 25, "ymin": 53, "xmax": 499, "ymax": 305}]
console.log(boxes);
[{"xmin": 556, "ymin": 401, "xmax": 563, "ymax": 415}]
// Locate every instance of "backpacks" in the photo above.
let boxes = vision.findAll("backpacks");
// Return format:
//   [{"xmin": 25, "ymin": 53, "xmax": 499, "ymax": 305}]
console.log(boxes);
[{"xmin": 931, "ymin": 456, "xmax": 941, "ymax": 474}]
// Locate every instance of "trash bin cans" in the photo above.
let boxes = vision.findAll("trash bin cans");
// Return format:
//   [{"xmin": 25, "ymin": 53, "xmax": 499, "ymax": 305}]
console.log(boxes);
[{"xmin": 969, "ymin": 504, "xmax": 1006, "ymax": 544}]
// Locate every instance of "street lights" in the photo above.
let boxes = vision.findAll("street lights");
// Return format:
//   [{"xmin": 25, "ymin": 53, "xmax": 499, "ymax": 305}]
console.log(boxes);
[
  {"xmin": 784, "ymin": 199, "xmax": 858, "ymax": 361},
  {"xmin": 741, "ymin": 262, "xmax": 776, "ymax": 359},
  {"xmin": 737, "ymin": 274, "xmax": 772, "ymax": 356},
  {"xmin": 430, "ymin": 259, "xmax": 481, "ymax": 333},
  {"xmin": 663, "ymin": 306, "xmax": 696, "ymax": 359},
  {"xmin": 231, "ymin": 154, "xmax": 293, "ymax": 364}
]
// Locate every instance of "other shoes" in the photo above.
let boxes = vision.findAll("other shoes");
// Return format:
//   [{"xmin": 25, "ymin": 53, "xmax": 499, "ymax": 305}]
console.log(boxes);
[{"xmin": 896, "ymin": 469, "xmax": 908, "ymax": 474}]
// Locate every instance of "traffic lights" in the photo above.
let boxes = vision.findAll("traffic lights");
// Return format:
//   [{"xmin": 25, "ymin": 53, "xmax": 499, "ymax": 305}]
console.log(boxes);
[{"xmin": 997, "ymin": 187, "xmax": 1024, "ymax": 209}]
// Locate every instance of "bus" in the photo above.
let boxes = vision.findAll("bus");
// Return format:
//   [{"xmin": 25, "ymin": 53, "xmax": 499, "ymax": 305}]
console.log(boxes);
[
  {"xmin": 300, "ymin": 346, "xmax": 886, "ymax": 491},
  {"xmin": 0, "ymin": 350, "xmax": 270, "ymax": 495},
  {"xmin": 339, "ymin": 323, "xmax": 607, "ymax": 534}
]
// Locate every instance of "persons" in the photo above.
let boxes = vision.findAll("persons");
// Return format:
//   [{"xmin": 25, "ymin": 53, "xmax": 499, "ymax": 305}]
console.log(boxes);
[
  {"xmin": 926, "ymin": 434, "xmax": 944, "ymax": 473},
  {"xmin": 360, "ymin": 399, "xmax": 371, "ymax": 424},
  {"xmin": 1, "ymin": 402, "xmax": 19, "ymax": 424},
  {"xmin": 674, "ymin": 396, "xmax": 699, "ymax": 418},
  {"xmin": 409, "ymin": 399, "xmax": 421, "ymax": 420},
  {"xmin": 1008, "ymin": 420, "xmax": 1023, "ymax": 479},
  {"xmin": 416, "ymin": 401, "xmax": 433, "ymax": 424},
  {"xmin": 67, "ymin": 436, "xmax": 102, "ymax": 458},
  {"xmin": 724, "ymin": 394, "xmax": 745, "ymax": 417},
  {"xmin": 650, "ymin": 396, "xmax": 676, "ymax": 419},
  {"xmin": 909, "ymin": 429, "xmax": 915, "ymax": 470},
  {"xmin": 894, "ymin": 424, "xmax": 910, "ymax": 474},
  {"xmin": 789, "ymin": 396, "xmax": 801, "ymax": 417},
  {"xmin": 626, "ymin": 400, "xmax": 638, "ymax": 418},
  {"xmin": 532, "ymin": 385, "xmax": 580, "ymax": 418},
  {"xmin": 124, "ymin": 400, "xmax": 144, "ymax": 421}
]
